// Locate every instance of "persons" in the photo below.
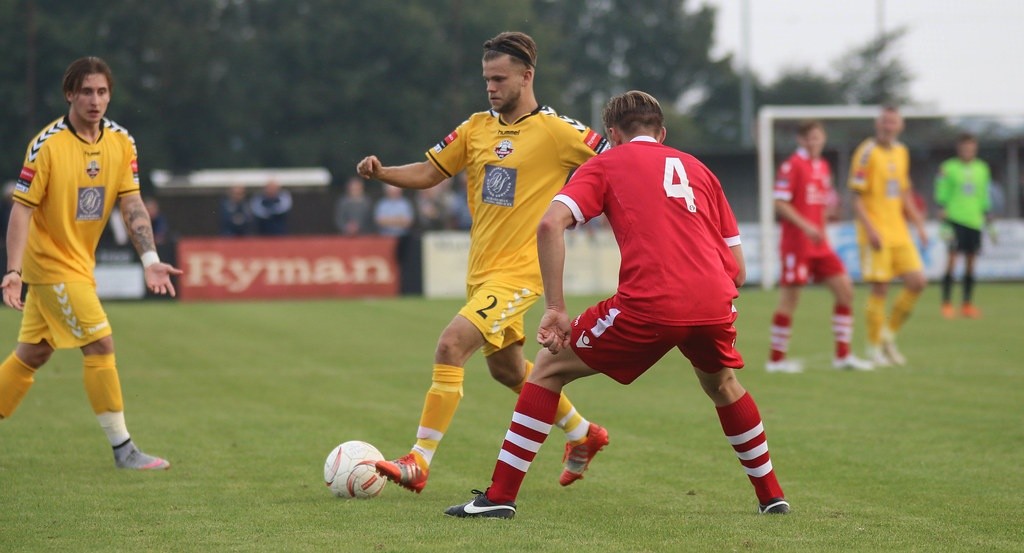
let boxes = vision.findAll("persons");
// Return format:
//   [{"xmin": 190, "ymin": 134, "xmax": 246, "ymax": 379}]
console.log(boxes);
[
  {"xmin": 145, "ymin": 198, "xmax": 169, "ymax": 298},
  {"xmin": 846, "ymin": 107, "xmax": 927, "ymax": 370},
  {"xmin": 0, "ymin": 56, "xmax": 182, "ymax": 472},
  {"xmin": 933, "ymin": 134, "xmax": 993, "ymax": 318},
  {"xmin": 221, "ymin": 180, "xmax": 292, "ymax": 234},
  {"xmin": 356, "ymin": 33, "xmax": 609, "ymax": 493},
  {"xmin": 765, "ymin": 121, "xmax": 873, "ymax": 372},
  {"xmin": 444, "ymin": 91, "xmax": 789, "ymax": 517},
  {"xmin": 333, "ymin": 173, "xmax": 471, "ymax": 295}
]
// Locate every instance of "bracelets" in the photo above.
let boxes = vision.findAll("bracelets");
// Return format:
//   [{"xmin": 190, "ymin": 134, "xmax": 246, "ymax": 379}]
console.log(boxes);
[
  {"xmin": 140, "ymin": 252, "xmax": 159, "ymax": 268},
  {"xmin": 7, "ymin": 270, "xmax": 21, "ymax": 277}
]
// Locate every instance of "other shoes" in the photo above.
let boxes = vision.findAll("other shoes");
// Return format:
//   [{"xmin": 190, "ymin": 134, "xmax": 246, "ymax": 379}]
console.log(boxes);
[
  {"xmin": 940, "ymin": 302, "xmax": 955, "ymax": 322},
  {"xmin": 833, "ymin": 357, "xmax": 871, "ymax": 374},
  {"xmin": 765, "ymin": 355, "xmax": 804, "ymax": 375},
  {"xmin": 959, "ymin": 303, "xmax": 979, "ymax": 319},
  {"xmin": 870, "ymin": 336, "xmax": 905, "ymax": 368}
]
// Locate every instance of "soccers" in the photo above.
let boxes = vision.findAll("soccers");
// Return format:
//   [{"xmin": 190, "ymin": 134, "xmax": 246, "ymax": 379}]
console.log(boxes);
[{"xmin": 323, "ymin": 439, "xmax": 388, "ymax": 498}]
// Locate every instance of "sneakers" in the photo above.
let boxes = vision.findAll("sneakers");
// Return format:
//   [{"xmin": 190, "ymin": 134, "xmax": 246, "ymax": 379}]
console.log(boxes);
[
  {"xmin": 757, "ymin": 498, "xmax": 788, "ymax": 517},
  {"xmin": 559, "ymin": 420, "xmax": 609, "ymax": 487},
  {"xmin": 112, "ymin": 442, "xmax": 168, "ymax": 470},
  {"xmin": 443, "ymin": 485, "xmax": 516, "ymax": 521},
  {"xmin": 374, "ymin": 453, "xmax": 429, "ymax": 495}
]
